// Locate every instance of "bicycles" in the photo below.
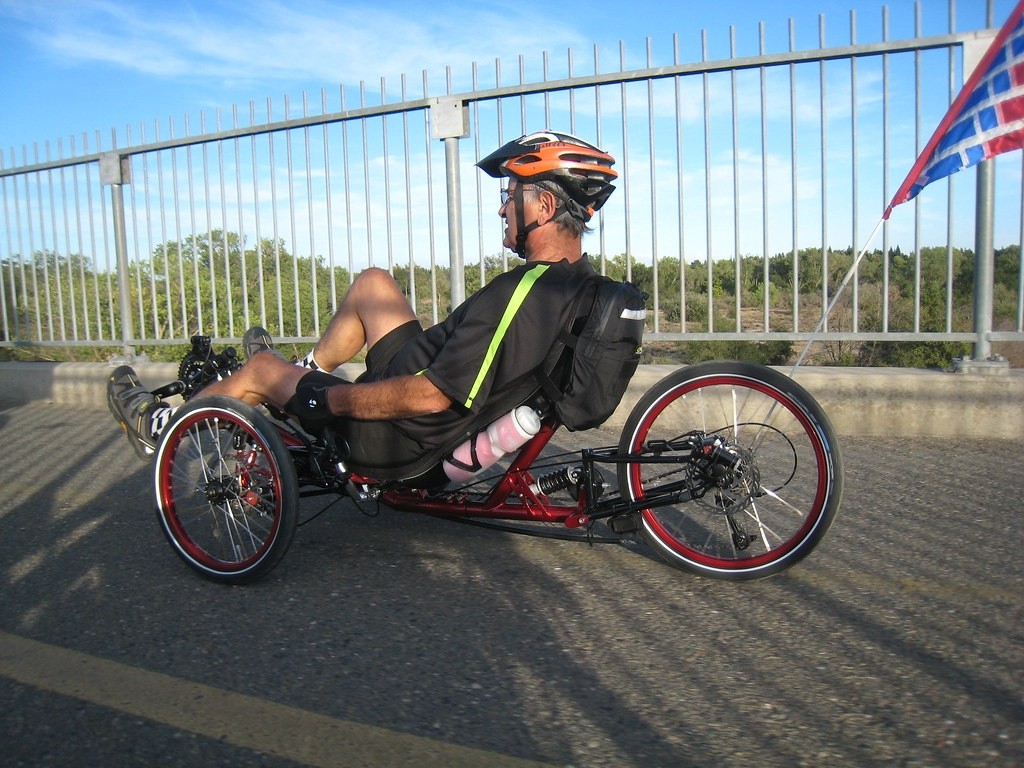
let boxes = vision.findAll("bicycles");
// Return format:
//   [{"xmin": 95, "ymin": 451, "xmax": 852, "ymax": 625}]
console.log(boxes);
[{"xmin": 136, "ymin": 324, "xmax": 844, "ymax": 588}]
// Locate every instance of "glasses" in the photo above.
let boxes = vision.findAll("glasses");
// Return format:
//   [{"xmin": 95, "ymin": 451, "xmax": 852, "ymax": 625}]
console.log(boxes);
[{"xmin": 500, "ymin": 188, "xmax": 534, "ymax": 204}]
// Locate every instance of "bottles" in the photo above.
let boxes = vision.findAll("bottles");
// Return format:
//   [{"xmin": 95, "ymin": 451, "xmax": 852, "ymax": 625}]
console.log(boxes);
[{"xmin": 443, "ymin": 405, "xmax": 543, "ymax": 484}]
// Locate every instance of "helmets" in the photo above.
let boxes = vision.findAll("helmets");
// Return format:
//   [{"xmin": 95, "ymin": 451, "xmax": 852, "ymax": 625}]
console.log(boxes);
[{"xmin": 473, "ymin": 131, "xmax": 619, "ymax": 222}]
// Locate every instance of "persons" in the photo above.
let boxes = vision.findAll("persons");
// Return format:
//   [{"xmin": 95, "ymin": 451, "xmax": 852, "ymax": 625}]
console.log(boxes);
[{"xmin": 107, "ymin": 131, "xmax": 649, "ymax": 466}]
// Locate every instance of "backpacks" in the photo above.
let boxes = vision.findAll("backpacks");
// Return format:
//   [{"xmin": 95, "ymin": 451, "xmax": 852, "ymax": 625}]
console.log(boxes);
[{"xmin": 532, "ymin": 275, "xmax": 649, "ymax": 433}]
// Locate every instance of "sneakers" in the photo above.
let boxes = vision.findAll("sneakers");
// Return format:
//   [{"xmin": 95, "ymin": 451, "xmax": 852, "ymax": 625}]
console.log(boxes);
[
  {"xmin": 107, "ymin": 365, "xmax": 171, "ymax": 462},
  {"xmin": 243, "ymin": 327, "xmax": 292, "ymax": 421}
]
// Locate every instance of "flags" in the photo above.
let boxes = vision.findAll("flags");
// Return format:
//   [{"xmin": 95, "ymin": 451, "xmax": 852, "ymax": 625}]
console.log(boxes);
[{"xmin": 882, "ymin": 0, "xmax": 1024, "ymax": 221}]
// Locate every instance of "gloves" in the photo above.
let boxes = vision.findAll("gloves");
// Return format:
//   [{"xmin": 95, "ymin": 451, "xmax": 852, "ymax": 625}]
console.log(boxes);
[{"xmin": 297, "ymin": 383, "xmax": 338, "ymax": 424}]
